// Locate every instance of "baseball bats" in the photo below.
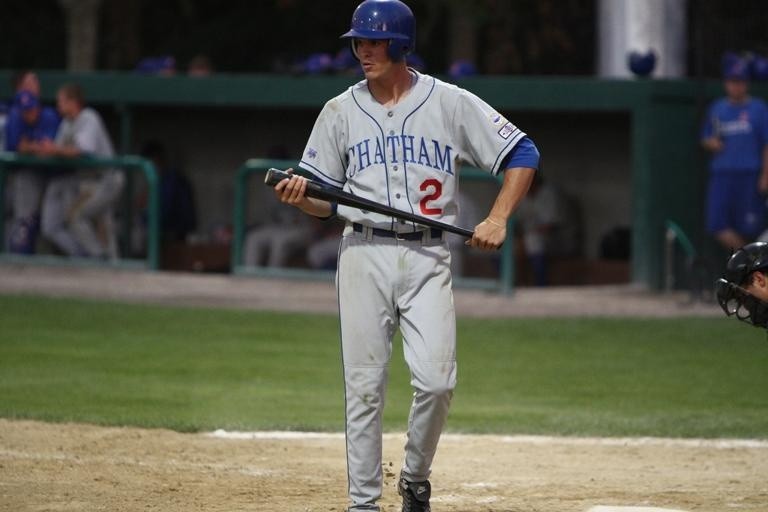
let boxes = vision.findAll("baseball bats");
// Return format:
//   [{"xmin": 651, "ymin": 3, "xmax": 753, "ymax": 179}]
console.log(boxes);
[{"xmin": 264, "ymin": 168, "xmax": 503, "ymax": 251}]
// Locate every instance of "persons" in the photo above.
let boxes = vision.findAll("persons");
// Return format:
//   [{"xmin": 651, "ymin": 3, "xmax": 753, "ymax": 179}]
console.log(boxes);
[
  {"xmin": 716, "ymin": 242, "xmax": 768, "ymax": 329},
  {"xmin": 274, "ymin": 1, "xmax": 540, "ymax": 512},
  {"xmin": 702, "ymin": 59, "xmax": 768, "ymax": 303},
  {"xmin": 5, "ymin": 72, "xmax": 126, "ymax": 258}
]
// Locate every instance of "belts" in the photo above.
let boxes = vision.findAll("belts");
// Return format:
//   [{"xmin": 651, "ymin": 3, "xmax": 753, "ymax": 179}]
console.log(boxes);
[{"xmin": 353, "ymin": 222, "xmax": 441, "ymax": 241}]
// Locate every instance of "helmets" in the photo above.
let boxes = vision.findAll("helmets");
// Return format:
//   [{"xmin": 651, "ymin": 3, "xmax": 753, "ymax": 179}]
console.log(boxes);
[
  {"xmin": 337, "ymin": 0, "xmax": 416, "ymax": 53},
  {"xmin": 716, "ymin": 241, "xmax": 768, "ymax": 301}
]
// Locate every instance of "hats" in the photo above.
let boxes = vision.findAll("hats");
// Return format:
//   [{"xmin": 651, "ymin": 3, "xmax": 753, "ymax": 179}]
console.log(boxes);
[{"xmin": 723, "ymin": 54, "xmax": 750, "ymax": 80}]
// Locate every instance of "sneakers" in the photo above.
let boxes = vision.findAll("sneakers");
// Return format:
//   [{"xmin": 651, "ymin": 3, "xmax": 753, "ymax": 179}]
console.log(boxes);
[{"xmin": 398, "ymin": 480, "xmax": 430, "ymax": 512}]
